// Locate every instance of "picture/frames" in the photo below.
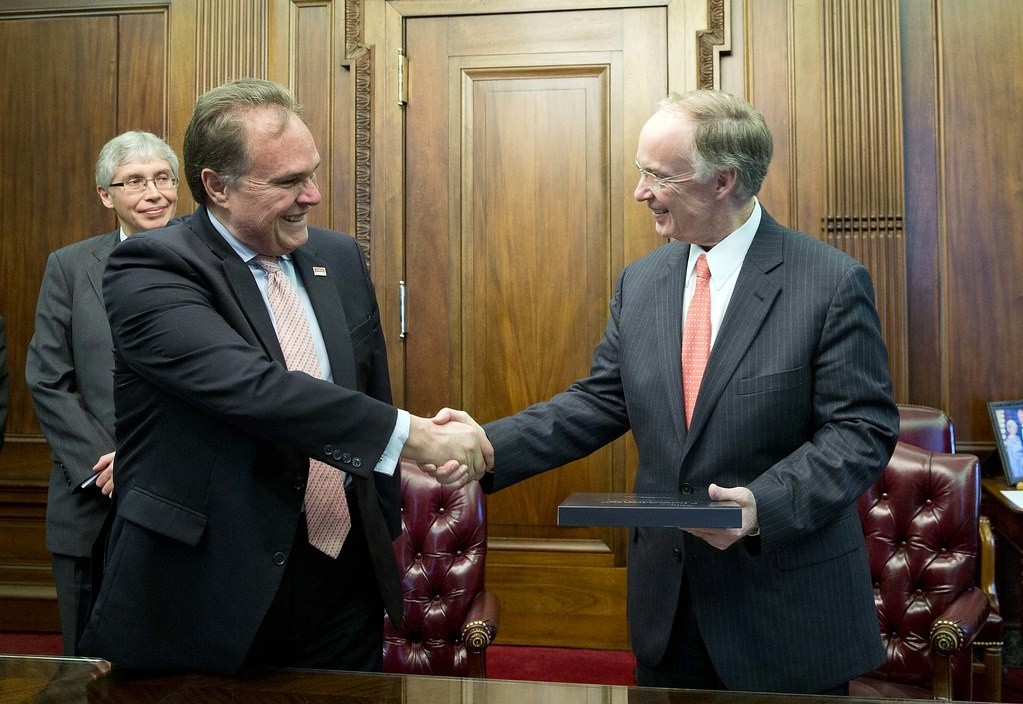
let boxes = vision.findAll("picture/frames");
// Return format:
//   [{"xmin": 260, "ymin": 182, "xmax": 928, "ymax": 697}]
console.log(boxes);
[{"xmin": 985, "ymin": 399, "xmax": 1023, "ymax": 486}]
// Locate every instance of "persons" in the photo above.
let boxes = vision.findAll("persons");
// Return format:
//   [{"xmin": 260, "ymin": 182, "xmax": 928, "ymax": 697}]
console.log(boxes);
[
  {"xmin": 25, "ymin": 131, "xmax": 180, "ymax": 657},
  {"xmin": 1005, "ymin": 408, "xmax": 1023, "ymax": 477},
  {"xmin": 79, "ymin": 76, "xmax": 494, "ymax": 671},
  {"xmin": 419, "ymin": 87, "xmax": 899, "ymax": 696}
]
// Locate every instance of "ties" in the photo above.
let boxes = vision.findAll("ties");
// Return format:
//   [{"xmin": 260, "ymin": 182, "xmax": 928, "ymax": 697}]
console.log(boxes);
[
  {"xmin": 255, "ymin": 254, "xmax": 351, "ymax": 560},
  {"xmin": 681, "ymin": 253, "xmax": 711, "ymax": 429}
]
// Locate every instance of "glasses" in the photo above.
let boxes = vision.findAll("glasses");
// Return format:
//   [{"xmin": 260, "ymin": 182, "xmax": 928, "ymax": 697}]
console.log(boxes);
[
  {"xmin": 107, "ymin": 174, "xmax": 181, "ymax": 192},
  {"xmin": 634, "ymin": 158, "xmax": 705, "ymax": 195}
]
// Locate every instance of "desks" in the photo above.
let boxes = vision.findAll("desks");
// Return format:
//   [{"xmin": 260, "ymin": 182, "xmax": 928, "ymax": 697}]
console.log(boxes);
[
  {"xmin": 0, "ymin": 654, "xmax": 942, "ymax": 704},
  {"xmin": 982, "ymin": 476, "xmax": 1023, "ymax": 566}
]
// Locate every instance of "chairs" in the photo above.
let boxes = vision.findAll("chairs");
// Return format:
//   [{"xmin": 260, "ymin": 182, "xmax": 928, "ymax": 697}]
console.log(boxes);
[
  {"xmin": 379, "ymin": 457, "xmax": 501, "ymax": 679},
  {"xmin": 890, "ymin": 402, "xmax": 1004, "ymax": 703},
  {"xmin": 848, "ymin": 442, "xmax": 991, "ymax": 701}
]
